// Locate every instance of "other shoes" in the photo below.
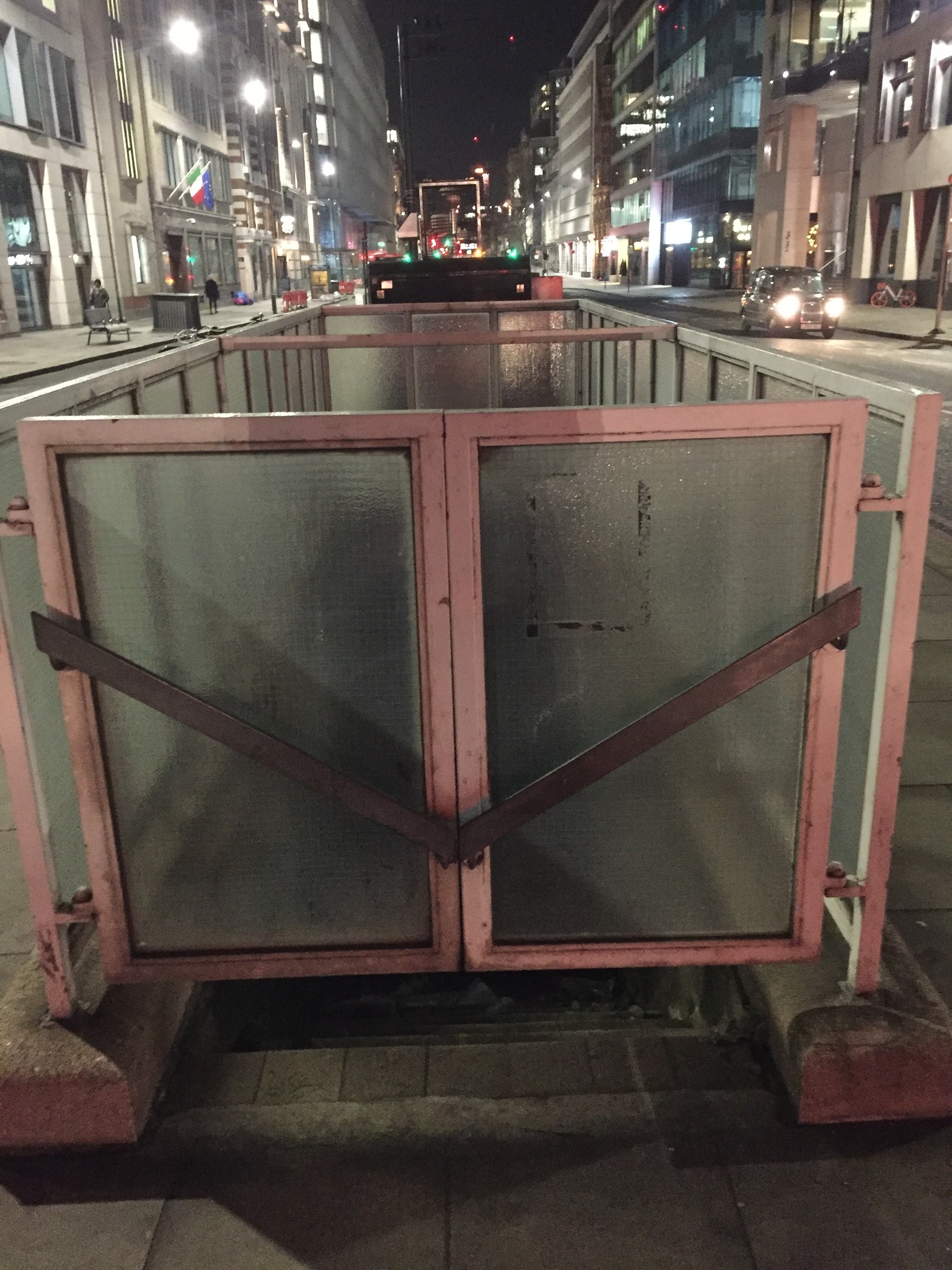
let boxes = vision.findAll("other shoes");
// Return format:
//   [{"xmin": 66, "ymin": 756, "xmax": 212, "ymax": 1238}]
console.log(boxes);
[
  {"xmin": 214, "ymin": 311, "xmax": 218, "ymax": 313},
  {"xmin": 209, "ymin": 313, "xmax": 213, "ymax": 315}
]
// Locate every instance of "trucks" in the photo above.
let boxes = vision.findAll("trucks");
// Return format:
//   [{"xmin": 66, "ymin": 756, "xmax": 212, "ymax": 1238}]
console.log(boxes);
[{"xmin": 361, "ymin": 178, "xmax": 533, "ymax": 304}]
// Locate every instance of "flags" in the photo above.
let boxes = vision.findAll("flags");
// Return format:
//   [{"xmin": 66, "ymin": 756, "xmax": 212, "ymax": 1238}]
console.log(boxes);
[
  {"xmin": 200, "ymin": 167, "xmax": 214, "ymax": 209},
  {"xmin": 187, "ymin": 164, "xmax": 205, "ymax": 205}
]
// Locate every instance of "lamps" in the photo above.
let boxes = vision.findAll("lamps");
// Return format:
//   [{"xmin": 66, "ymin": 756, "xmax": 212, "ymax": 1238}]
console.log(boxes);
[
  {"xmin": 225, "ymin": 222, "xmax": 236, "ymax": 229},
  {"xmin": 186, "ymin": 217, "xmax": 196, "ymax": 224}
]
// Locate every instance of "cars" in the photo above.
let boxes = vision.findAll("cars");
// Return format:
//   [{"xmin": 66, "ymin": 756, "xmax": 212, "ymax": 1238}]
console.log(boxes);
[{"xmin": 738, "ymin": 265, "xmax": 842, "ymax": 340}]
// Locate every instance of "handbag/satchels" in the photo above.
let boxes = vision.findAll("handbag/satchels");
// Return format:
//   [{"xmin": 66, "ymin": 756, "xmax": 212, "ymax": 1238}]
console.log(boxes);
[
  {"xmin": 90, "ymin": 290, "xmax": 95, "ymax": 306},
  {"xmin": 103, "ymin": 300, "xmax": 112, "ymax": 320},
  {"xmin": 213, "ymin": 292, "xmax": 220, "ymax": 302}
]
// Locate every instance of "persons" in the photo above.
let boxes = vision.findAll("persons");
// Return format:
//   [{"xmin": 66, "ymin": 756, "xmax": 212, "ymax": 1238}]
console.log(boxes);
[
  {"xmin": 204, "ymin": 274, "xmax": 219, "ymax": 315},
  {"xmin": 88, "ymin": 278, "xmax": 110, "ymax": 309}
]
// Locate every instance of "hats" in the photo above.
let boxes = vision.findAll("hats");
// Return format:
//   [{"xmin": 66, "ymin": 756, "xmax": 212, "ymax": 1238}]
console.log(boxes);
[{"xmin": 94, "ymin": 279, "xmax": 101, "ymax": 285}]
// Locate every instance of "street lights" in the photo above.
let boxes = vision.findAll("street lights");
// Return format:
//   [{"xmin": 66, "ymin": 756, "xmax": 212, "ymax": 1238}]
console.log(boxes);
[{"xmin": 475, "ymin": 168, "xmax": 515, "ymax": 254}]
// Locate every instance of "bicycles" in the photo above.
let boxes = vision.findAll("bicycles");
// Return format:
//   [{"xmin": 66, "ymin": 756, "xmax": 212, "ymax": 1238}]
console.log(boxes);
[{"xmin": 870, "ymin": 277, "xmax": 916, "ymax": 309}]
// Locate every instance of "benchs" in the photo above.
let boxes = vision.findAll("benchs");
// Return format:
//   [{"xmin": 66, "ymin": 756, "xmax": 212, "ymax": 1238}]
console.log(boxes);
[{"xmin": 82, "ymin": 304, "xmax": 132, "ymax": 345}]
